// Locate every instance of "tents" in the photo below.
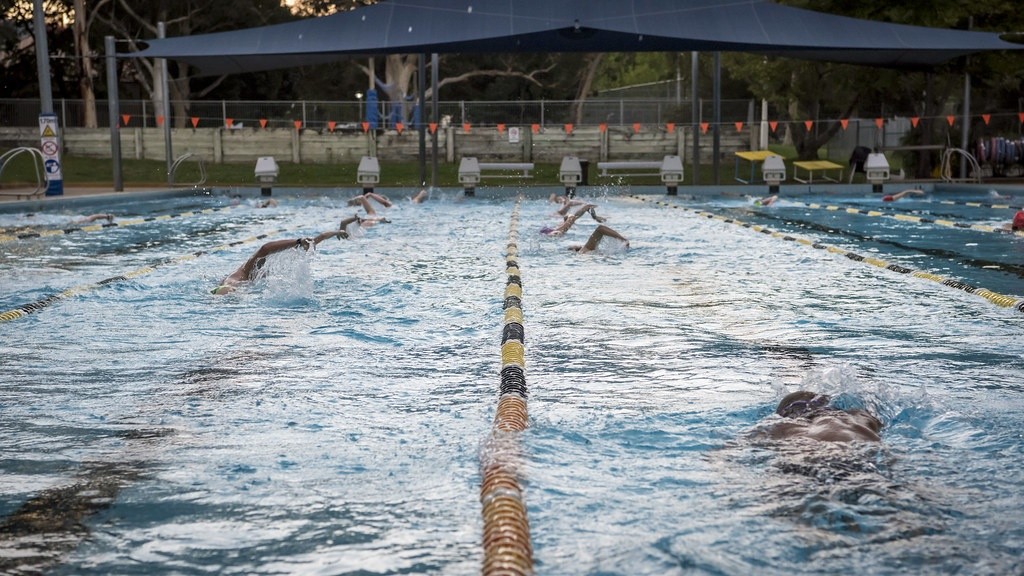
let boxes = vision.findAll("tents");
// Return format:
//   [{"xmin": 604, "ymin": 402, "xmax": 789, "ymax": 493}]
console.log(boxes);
[{"xmin": 111, "ymin": 0, "xmax": 1024, "ymax": 183}]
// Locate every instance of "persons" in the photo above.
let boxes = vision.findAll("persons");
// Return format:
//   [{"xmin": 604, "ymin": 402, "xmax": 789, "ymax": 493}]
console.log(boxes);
[
  {"xmin": 760, "ymin": 391, "xmax": 881, "ymax": 441},
  {"xmin": 366, "ymin": 193, "xmax": 392, "ymax": 207},
  {"xmin": 75, "ymin": 214, "xmax": 113, "ymax": 223},
  {"xmin": 550, "ymin": 192, "xmax": 564, "ymax": 203},
  {"xmin": 541, "ymin": 214, "xmax": 576, "ymax": 234},
  {"xmin": 315, "ymin": 230, "xmax": 349, "ymax": 244},
  {"xmin": 340, "ymin": 216, "xmax": 361, "ymax": 231},
  {"xmin": 883, "ymin": 190, "xmax": 924, "ymax": 201},
  {"xmin": 1004, "ymin": 211, "xmax": 1024, "ymax": 231},
  {"xmin": 555, "ymin": 201, "xmax": 582, "ymax": 216},
  {"xmin": 564, "ymin": 204, "xmax": 606, "ymax": 223},
  {"xmin": 256, "ymin": 199, "xmax": 270, "ymax": 209},
  {"xmin": 349, "ymin": 195, "xmax": 377, "ymax": 226},
  {"xmin": 211, "ymin": 237, "xmax": 316, "ymax": 295},
  {"xmin": 568, "ymin": 225, "xmax": 630, "ymax": 255},
  {"xmin": 755, "ymin": 195, "xmax": 778, "ymax": 206},
  {"xmin": 411, "ymin": 190, "xmax": 427, "ymax": 203}
]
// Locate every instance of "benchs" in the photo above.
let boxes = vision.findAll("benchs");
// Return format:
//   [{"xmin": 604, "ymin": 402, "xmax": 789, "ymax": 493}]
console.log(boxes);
[
  {"xmin": 479, "ymin": 163, "xmax": 534, "ymax": 179},
  {"xmin": 598, "ymin": 161, "xmax": 662, "ymax": 176}
]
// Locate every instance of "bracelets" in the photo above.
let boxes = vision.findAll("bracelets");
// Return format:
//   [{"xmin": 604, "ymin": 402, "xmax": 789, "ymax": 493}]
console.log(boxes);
[
  {"xmin": 297, "ymin": 238, "xmax": 302, "ymax": 244},
  {"xmin": 107, "ymin": 215, "xmax": 109, "ymax": 220}
]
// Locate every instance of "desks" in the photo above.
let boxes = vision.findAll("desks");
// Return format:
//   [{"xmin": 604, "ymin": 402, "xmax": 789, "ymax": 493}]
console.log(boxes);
[
  {"xmin": 733, "ymin": 151, "xmax": 787, "ymax": 183},
  {"xmin": 792, "ymin": 161, "xmax": 845, "ymax": 184}
]
